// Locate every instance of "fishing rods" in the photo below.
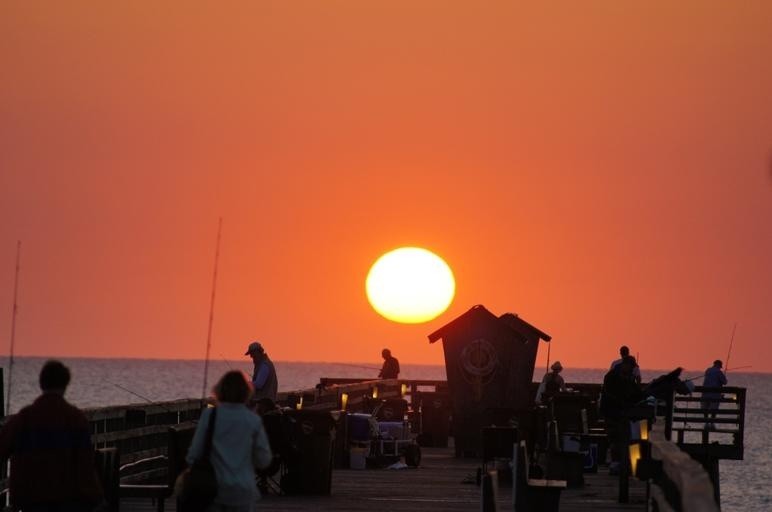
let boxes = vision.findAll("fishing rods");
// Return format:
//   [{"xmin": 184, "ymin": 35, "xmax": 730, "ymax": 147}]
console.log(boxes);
[
  {"xmin": 725, "ymin": 324, "xmax": 736, "ymax": 376},
  {"xmin": 330, "ymin": 362, "xmax": 380, "ymax": 370},
  {"xmin": 545, "ymin": 342, "xmax": 552, "ymax": 370},
  {"xmin": 682, "ymin": 365, "xmax": 752, "ymax": 382},
  {"xmin": 115, "ymin": 383, "xmax": 199, "ymax": 427},
  {"xmin": 6, "ymin": 242, "xmax": 20, "ymax": 412}
]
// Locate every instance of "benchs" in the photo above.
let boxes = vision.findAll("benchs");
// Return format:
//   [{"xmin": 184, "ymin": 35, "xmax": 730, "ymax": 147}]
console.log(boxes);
[{"xmin": 119, "ymin": 406, "xmax": 291, "ymax": 512}]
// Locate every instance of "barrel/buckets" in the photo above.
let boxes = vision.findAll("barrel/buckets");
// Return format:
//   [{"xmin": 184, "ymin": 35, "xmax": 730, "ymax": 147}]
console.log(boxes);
[
  {"xmin": 564, "ymin": 432, "xmax": 581, "ymax": 453},
  {"xmin": 377, "ymin": 420, "xmax": 403, "ymax": 438},
  {"xmin": 347, "ymin": 413, "xmax": 372, "ymax": 443},
  {"xmin": 348, "ymin": 448, "xmax": 366, "ymax": 471}
]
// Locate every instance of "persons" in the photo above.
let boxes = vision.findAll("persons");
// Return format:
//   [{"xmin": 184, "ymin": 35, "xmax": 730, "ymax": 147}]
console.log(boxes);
[
  {"xmin": 253, "ymin": 394, "xmax": 290, "ymax": 432},
  {"xmin": 611, "ymin": 345, "xmax": 638, "ymax": 371},
  {"xmin": 244, "ymin": 342, "xmax": 277, "ymax": 405},
  {"xmin": 377, "ymin": 349, "xmax": 400, "ymax": 381},
  {"xmin": 700, "ymin": 360, "xmax": 727, "ymax": 430},
  {"xmin": 183, "ymin": 368, "xmax": 272, "ymax": 512},
  {"xmin": 599, "ymin": 354, "xmax": 640, "ymax": 432},
  {"xmin": 1, "ymin": 354, "xmax": 112, "ymax": 512},
  {"xmin": 534, "ymin": 361, "xmax": 568, "ymax": 415}
]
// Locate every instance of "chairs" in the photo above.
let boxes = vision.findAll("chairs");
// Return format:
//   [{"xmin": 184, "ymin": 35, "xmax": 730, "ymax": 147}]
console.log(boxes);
[{"xmin": 482, "ymin": 409, "xmax": 606, "ymax": 512}]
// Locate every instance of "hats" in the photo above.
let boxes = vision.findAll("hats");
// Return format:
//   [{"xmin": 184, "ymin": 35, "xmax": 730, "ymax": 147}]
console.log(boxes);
[{"xmin": 245, "ymin": 343, "xmax": 261, "ymax": 355}]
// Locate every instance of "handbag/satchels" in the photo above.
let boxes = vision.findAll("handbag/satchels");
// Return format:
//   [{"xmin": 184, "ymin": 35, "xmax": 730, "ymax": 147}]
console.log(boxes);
[{"xmin": 177, "ymin": 462, "xmax": 218, "ymax": 512}]
